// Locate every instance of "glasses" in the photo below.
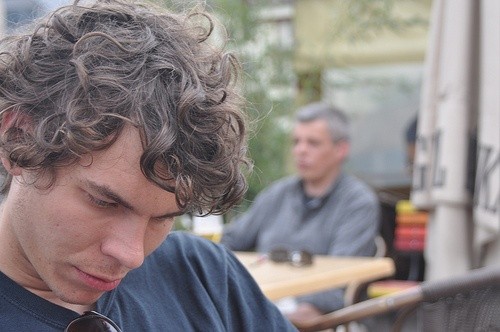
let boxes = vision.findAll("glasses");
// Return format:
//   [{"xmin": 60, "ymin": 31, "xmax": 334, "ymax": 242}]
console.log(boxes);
[
  {"xmin": 64, "ymin": 310, "xmax": 121, "ymax": 332},
  {"xmin": 252, "ymin": 248, "xmax": 312, "ymax": 267}
]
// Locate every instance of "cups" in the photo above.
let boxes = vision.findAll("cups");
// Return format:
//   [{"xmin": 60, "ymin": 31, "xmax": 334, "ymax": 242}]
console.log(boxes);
[{"xmin": 193, "ymin": 210, "xmax": 222, "ymax": 244}]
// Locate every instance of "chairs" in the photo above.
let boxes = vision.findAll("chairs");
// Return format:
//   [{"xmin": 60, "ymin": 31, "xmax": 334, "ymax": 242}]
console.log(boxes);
[{"xmin": 317, "ymin": 234, "xmax": 387, "ymax": 332}]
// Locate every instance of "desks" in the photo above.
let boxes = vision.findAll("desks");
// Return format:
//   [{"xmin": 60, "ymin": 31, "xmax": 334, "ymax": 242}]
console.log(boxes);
[{"xmin": 231, "ymin": 252, "xmax": 396, "ymax": 306}]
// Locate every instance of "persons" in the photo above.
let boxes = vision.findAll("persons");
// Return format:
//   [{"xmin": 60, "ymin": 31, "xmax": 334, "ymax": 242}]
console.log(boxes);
[
  {"xmin": 0, "ymin": 0, "xmax": 298, "ymax": 332},
  {"xmin": 217, "ymin": 101, "xmax": 381, "ymax": 332}
]
